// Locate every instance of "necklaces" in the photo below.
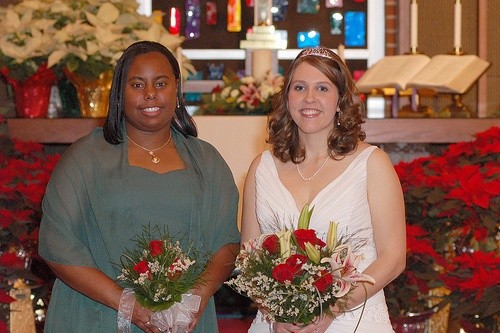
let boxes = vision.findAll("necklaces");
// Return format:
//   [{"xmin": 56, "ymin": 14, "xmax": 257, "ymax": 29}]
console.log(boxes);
[
  {"xmin": 128, "ymin": 130, "xmax": 172, "ymax": 164},
  {"xmin": 295, "ymin": 150, "xmax": 333, "ymax": 181}
]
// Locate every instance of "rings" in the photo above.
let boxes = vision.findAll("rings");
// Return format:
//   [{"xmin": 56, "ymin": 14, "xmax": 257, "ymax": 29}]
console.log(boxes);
[
  {"xmin": 144, "ymin": 321, "xmax": 150, "ymax": 325},
  {"xmin": 315, "ymin": 329, "xmax": 320, "ymax": 332}
]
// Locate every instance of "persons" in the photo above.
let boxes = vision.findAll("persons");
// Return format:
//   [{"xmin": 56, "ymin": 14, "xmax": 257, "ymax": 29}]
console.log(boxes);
[
  {"xmin": 38, "ymin": 40, "xmax": 241, "ymax": 333},
  {"xmin": 241, "ymin": 48, "xmax": 407, "ymax": 333}
]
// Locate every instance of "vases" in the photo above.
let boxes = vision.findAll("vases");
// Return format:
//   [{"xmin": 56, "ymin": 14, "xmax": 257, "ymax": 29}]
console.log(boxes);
[
  {"xmin": 63, "ymin": 65, "xmax": 113, "ymax": 117},
  {"xmin": 1, "ymin": 62, "xmax": 61, "ymax": 118}
]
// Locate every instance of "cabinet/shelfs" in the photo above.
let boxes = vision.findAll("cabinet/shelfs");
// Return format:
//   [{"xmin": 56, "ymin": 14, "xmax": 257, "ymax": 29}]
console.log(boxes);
[{"xmin": 137, "ymin": 0, "xmax": 385, "ymax": 115}]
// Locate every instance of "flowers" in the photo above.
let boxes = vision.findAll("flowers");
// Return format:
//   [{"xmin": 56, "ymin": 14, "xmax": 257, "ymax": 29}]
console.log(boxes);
[
  {"xmin": 393, "ymin": 126, "xmax": 500, "ymax": 333},
  {"xmin": 0, "ymin": 133, "xmax": 84, "ymax": 333},
  {"xmin": 0, "ymin": 0, "xmax": 284, "ymax": 116},
  {"xmin": 223, "ymin": 201, "xmax": 375, "ymax": 333},
  {"xmin": 109, "ymin": 220, "xmax": 213, "ymax": 313}
]
